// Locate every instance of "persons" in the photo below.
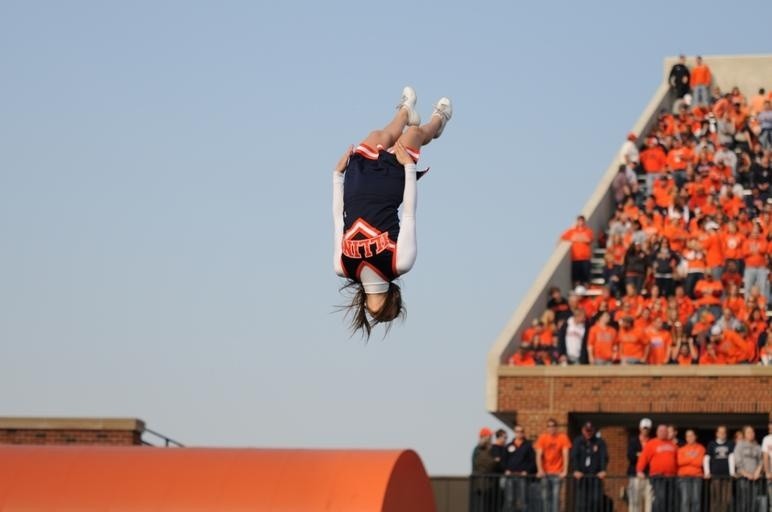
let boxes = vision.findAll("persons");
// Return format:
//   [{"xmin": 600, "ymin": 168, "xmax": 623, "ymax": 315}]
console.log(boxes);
[
  {"xmin": 472, "ymin": 418, "xmax": 771, "ymax": 511},
  {"xmin": 506, "ymin": 54, "xmax": 771, "ymax": 368},
  {"xmin": 332, "ymin": 86, "xmax": 453, "ymax": 337}
]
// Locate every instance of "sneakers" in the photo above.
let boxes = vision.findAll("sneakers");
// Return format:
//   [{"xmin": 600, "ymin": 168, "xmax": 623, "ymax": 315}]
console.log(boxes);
[
  {"xmin": 398, "ymin": 87, "xmax": 421, "ymax": 127},
  {"xmin": 430, "ymin": 98, "xmax": 451, "ymax": 139}
]
